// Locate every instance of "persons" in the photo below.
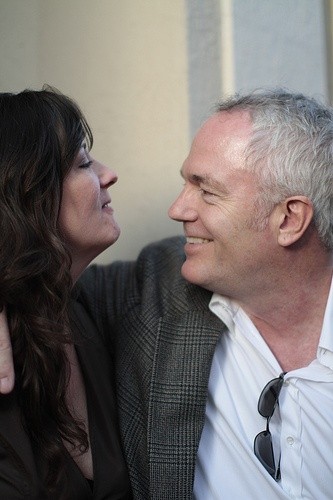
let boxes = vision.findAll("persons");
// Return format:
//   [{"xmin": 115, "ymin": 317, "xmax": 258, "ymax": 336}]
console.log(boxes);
[
  {"xmin": 0, "ymin": 85, "xmax": 133, "ymax": 500},
  {"xmin": 0, "ymin": 91, "xmax": 332, "ymax": 500}
]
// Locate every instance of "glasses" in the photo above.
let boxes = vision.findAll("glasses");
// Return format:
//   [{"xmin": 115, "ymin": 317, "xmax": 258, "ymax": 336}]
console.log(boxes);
[{"xmin": 254, "ymin": 372, "xmax": 286, "ymax": 482}]
